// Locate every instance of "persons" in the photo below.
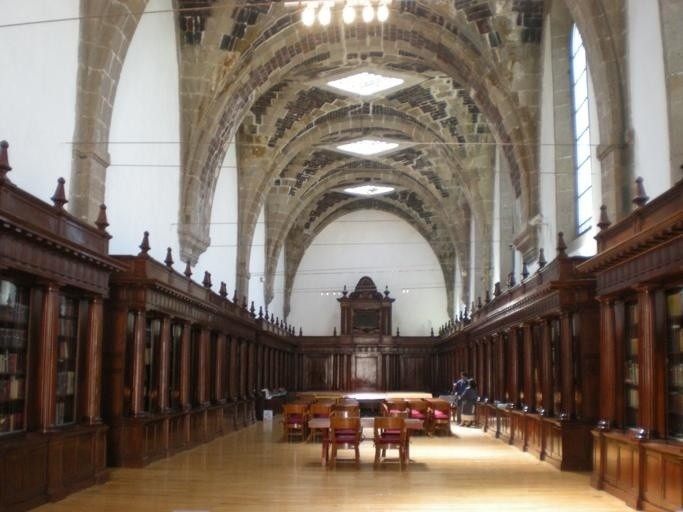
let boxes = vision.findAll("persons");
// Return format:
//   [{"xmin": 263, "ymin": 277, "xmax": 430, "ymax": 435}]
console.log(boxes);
[
  {"xmin": 457, "ymin": 378, "xmax": 478, "ymax": 427},
  {"xmin": 453, "ymin": 371, "xmax": 468, "ymax": 425},
  {"xmin": 450, "ymin": 383, "xmax": 458, "ymax": 421}
]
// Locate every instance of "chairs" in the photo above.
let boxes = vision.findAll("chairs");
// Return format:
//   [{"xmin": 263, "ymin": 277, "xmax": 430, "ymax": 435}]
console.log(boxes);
[{"xmin": 282, "ymin": 395, "xmax": 451, "ymax": 474}]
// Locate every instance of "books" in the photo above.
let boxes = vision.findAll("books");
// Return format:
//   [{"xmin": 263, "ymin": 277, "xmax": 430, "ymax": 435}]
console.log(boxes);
[
  {"xmin": 624, "ymin": 291, "xmax": 683, "ymax": 435},
  {"xmin": 0, "ymin": 281, "xmax": 78, "ymax": 432}
]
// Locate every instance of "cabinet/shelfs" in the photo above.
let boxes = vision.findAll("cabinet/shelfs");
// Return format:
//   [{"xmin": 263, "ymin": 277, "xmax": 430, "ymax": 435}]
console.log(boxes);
[
  {"xmin": 576, "ymin": 222, "xmax": 683, "ymax": 512},
  {"xmin": 108, "ymin": 231, "xmax": 294, "ymax": 469},
  {"xmin": 0, "ymin": 137, "xmax": 109, "ymax": 510},
  {"xmin": 434, "ymin": 232, "xmax": 591, "ymax": 469}
]
